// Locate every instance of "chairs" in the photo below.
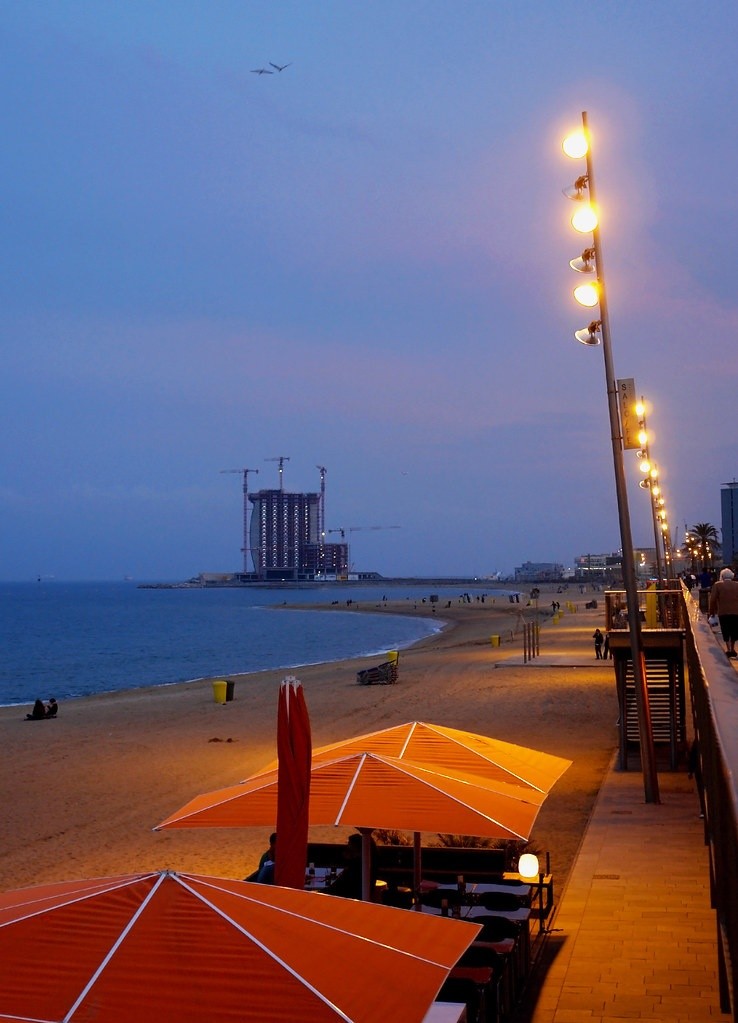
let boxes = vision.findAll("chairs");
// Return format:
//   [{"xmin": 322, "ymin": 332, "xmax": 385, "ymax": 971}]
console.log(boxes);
[
  {"xmin": 478, "ymin": 891, "xmax": 526, "ymax": 976},
  {"xmin": 471, "ymin": 915, "xmax": 520, "ymax": 1000},
  {"xmin": 456, "ymin": 945, "xmax": 504, "ymax": 988}
]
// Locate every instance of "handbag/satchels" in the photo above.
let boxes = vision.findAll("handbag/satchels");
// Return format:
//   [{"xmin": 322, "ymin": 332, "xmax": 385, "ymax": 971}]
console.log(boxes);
[{"xmin": 708, "ymin": 617, "xmax": 719, "ymax": 627}]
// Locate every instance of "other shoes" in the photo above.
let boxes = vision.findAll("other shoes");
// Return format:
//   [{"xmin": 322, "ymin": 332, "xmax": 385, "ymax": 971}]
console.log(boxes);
[{"xmin": 725, "ymin": 650, "xmax": 737, "ymax": 659}]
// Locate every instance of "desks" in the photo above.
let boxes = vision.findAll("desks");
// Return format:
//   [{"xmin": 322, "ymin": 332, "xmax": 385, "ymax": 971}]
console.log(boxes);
[
  {"xmin": 410, "ymin": 904, "xmax": 471, "ymax": 919},
  {"xmin": 438, "ymin": 883, "xmax": 473, "ymax": 901},
  {"xmin": 467, "ymin": 906, "xmax": 531, "ymax": 973},
  {"xmin": 472, "ymin": 938, "xmax": 516, "ymax": 1014},
  {"xmin": 472, "ymin": 883, "xmax": 533, "ymax": 908},
  {"xmin": 505, "ymin": 872, "xmax": 554, "ymax": 908},
  {"xmin": 305, "ymin": 867, "xmax": 344, "ymax": 892},
  {"xmin": 450, "ymin": 965, "xmax": 498, "ymax": 1023}
]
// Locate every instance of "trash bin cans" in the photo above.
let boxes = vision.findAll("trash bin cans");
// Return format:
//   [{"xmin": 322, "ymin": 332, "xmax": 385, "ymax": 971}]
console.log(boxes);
[
  {"xmin": 212, "ymin": 680, "xmax": 235, "ymax": 705},
  {"xmin": 388, "ymin": 651, "xmax": 399, "ymax": 665},
  {"xmin": 491, "ymin": 635, "xmax": 501, "ymax": 647}
]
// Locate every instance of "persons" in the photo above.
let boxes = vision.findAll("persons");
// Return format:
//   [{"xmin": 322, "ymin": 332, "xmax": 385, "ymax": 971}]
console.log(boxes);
[
  {"xmin": 26, "ymin": 699, "xmax": 45, "ymax": 719},
  {"xmin": 593, "ymin": 629, "xmax": 603, "ymax": 660},
  {"xmin": 603, "ymin": 632, "xmax": 613, "ymax": 660},
  {"xmin": 335, "ymin": 834, "xmax": 363, "ymax": 899},
  {"xmin": 45, "ymin": 697, "xmax": 58, "ymax": 718},
  {"xmin": 709, "ymin": 568, "xmax": 738, "ymax": 658},
  {"xmin": 550, "ymin": 601, "xmax": 560, "ymax": 612},
  {"xmin": 699, "ymin": 567, "xmax": 717, "ymax": 588},
  {"xmin": 257, "ymin": 833, "xmax": 277, "ymax": 884}
]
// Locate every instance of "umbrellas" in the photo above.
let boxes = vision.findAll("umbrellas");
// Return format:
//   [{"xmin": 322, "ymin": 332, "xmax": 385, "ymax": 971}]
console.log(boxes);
[
  {"xmin": 152, "ymin": 720, "xmax": 574, "ymax": 912},
  {"xmin": 0, "ymin": 869, "xmax": 484, "ymax": 1023}
]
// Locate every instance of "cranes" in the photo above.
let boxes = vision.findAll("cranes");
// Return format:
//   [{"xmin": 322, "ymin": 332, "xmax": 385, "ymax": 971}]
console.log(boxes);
[
  {"xmin": 329, "ymin": 524, "xmax": 401, "ymax": 545},
  {"xmin": 220, "ymin": 468, "xmax": 258, "ymax": 572},
  {"xmin": 314, "ymin": 464, "xmax": 327, "ymax": 543},
  {"xmin": 264, "ymin": 456, "xmax": 290, "ymax": 492}
]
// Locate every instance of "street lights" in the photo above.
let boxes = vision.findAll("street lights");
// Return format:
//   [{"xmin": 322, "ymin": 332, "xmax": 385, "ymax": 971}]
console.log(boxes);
[
  {"xmin": 564, "ymin": 111, "xmax": 659, "ymax": 805},
  {"xmin": 651, "ymin": 462, "xmax": 676, "ymax": 589},
  {"xmin": 635, "ymin": 395, "xmax": 669, "ymax": 629}
]
